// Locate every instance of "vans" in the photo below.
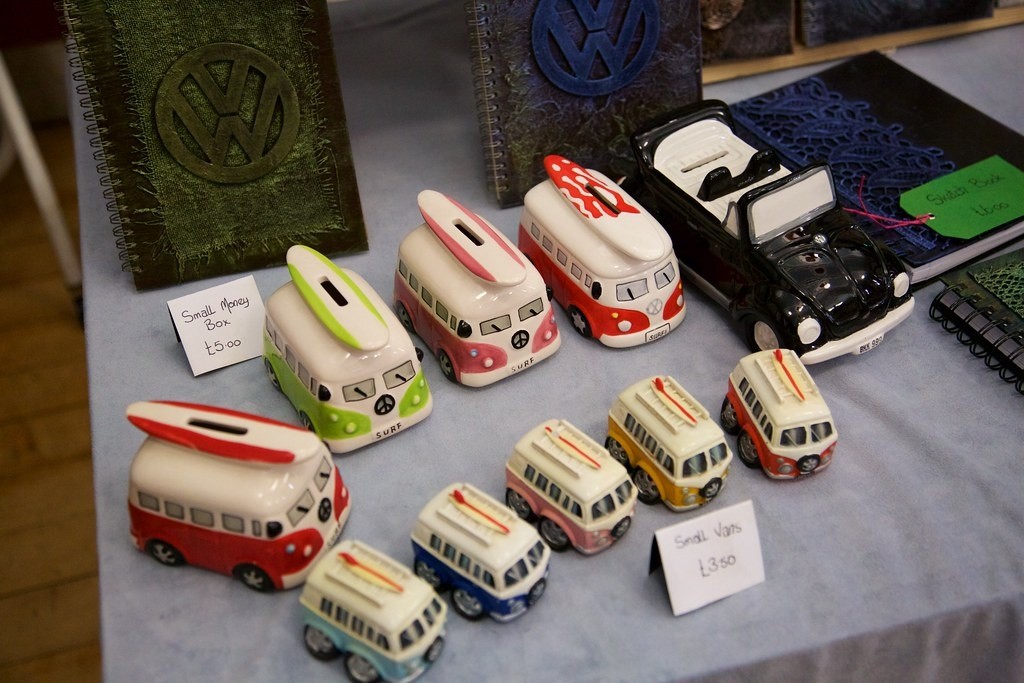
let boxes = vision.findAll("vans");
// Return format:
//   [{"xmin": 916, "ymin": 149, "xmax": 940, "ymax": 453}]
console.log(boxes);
[
  {"xmin": 297, "ymin": 540, "xmax": 446, "ymax": 683},
  {"xmin": 720, "ymin": 347, "xmax": 838, "ymax": 483},
  {"xmin": 605, "ymin": 374, "xmax": 734, "ymax": 512},
  {"xmin": 504, "ymin": 417, "xmax": 637, "ymax": 555},
  {"xmin": 412, "ymin": 481, "xmax": 549, "ymax": 622}
]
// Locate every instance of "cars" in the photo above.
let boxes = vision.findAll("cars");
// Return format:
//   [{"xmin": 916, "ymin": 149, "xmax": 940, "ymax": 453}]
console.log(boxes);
[{"xmin": 633, "ymin": 98, "xmax": 916, "ymax": 365}]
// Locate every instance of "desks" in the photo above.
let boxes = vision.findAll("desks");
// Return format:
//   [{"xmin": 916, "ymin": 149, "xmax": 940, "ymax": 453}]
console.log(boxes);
[{"xmin": 64, "ymin": 0, "xmax": 1024, "ymax": 683}]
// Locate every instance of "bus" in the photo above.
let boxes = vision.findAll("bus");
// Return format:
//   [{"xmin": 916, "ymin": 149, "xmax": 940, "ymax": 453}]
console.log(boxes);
[
  {"xmin": 262, "ymin": 245, "xmax": 434, "ymax": 452},
  {"xmin": 394, "ymin": 189, "xmax": 562, "ymax": 389},
  {"xmin": 517, "ymin": 155, "xmax": 686, "ymax": 351},
  {"xmin": 125, "ymin": 399, "xmax": 351, "ymax": 593}
]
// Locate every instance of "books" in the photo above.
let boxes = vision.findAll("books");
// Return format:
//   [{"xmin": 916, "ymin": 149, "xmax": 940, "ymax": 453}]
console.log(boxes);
[
  {"xmin": 462, "ymin": 0, "xmax": 702, "ymax": 211},
  {"xmin": 929, "ymin": 245, "xmax": 1024, "ymax": 400},
  {"xmin": 55, "ymin": 0, "xmax": 368, "ymax": 291},
  {"xmin": 701, "ymin": 0, "xmax": 1024, "ymax": 65},
  {"xmin": 730, "ymin": 48, "xmax": 1024, "ymax": 288}
]
